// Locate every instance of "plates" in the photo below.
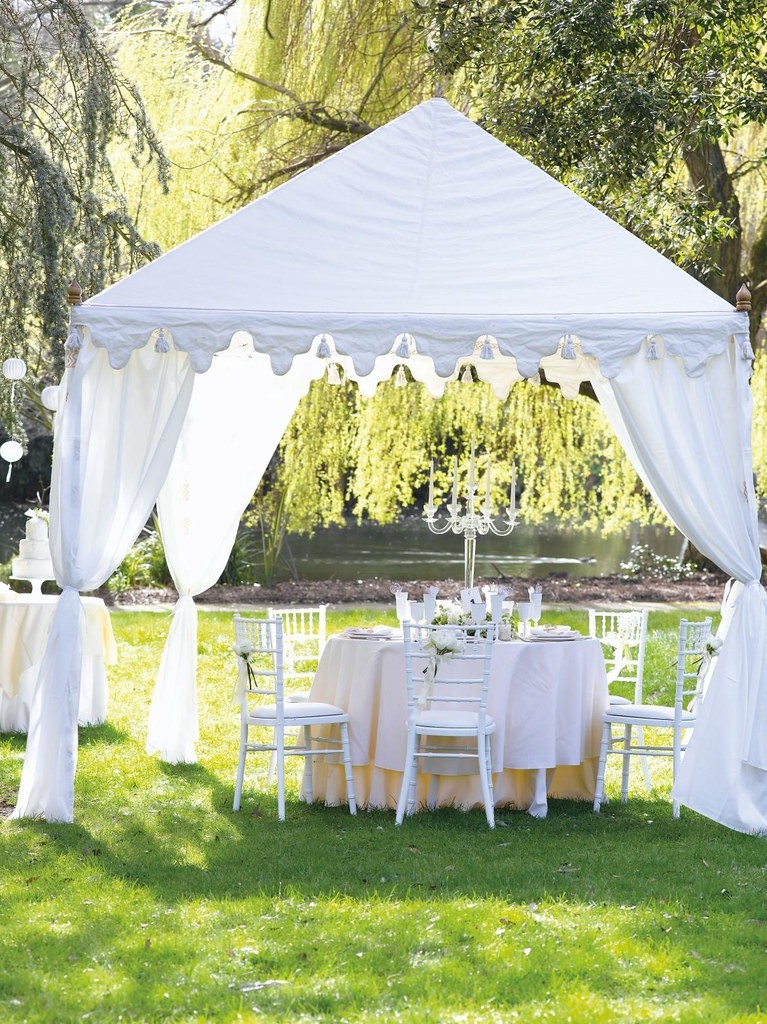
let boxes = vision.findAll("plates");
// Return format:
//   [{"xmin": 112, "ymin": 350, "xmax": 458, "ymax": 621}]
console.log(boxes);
[
  {"xmin": 345, "ymin": 632, "xmax": 402, "ymax": 639},
  {"xmin": 531, "ymin": 635, "xmax": 576, "ymax": 641}
]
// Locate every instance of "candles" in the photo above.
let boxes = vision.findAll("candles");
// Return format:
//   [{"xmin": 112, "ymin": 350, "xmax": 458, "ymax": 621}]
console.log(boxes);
[
  {"xmin": 454, "ymin": 455, "xmax": 457, "ymax": 504},
  {"xmin": 510, "ymin": 460, "xmax": 515, "ymax": 509},
  {"xmin": 470, "ymin": 436, "xmax": 474, "ymax": 493},
  {"xmin": 429, "ymin": 457, "xmax": 433, "ymax": 510},
  {"xmin": 486, "ymin": 460, "xmax": 490, "ymax": 509}
]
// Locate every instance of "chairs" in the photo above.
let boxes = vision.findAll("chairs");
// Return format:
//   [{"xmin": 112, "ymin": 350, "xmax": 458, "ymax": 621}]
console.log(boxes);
[
  {"xmin": 266, "ymin": 605, "xmax": 327, "ymax": 786},
  {"xmin": 589, "ymin": 608, "xmax": 652, "ymax": 794},
  {"xmin": 395, "ymin": 620, "xmax": 497, "ymax": 830},
  {"xmin": 231, "ymin": 610, "xmax": 358, "ymax": 823},
  {"xmin": 593, "ymin": 616, "xmax": 713, "ymax": 819}
]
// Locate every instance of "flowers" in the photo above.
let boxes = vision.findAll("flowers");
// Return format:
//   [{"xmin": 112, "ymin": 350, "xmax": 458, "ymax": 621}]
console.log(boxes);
[
  {"xmin": 605, "ymin": 611, "xmax": 638, "ymax": 674},
  {"xmin": 434, "ymin": 604, "xmax": 514, "ymax": 640},
  {"xmin": 24, "ymin": 508, "xmax": 50, "ymax": 526},
  {"xmin": 691, "ymin": 635, "xmax": 723, "ymax": 676},
  {"xmin": 422, "ymin": 632, "xmax": 466, "ymax": 679},
  {"xmin": 232, "ymin": 639, "xmax": 257, "ymax": 690}
]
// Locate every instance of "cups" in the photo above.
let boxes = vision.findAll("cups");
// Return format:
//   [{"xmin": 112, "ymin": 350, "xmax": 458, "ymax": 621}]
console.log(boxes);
[
  {"xmin": 499, "ymin": 624, "xmax": 512, "ymax": 640},
  {"xmin": 518, "ymin": 622, "xmax": 531, "ymax": 635}
]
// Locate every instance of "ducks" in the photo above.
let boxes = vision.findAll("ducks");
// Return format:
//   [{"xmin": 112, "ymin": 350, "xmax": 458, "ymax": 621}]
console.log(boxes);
[{"xmin": 581, "ymin": 554, "xmax": 596, "ymax": 563}]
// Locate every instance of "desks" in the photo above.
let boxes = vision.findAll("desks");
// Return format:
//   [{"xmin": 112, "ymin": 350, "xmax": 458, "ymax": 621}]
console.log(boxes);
[
  {"xmin": 0, "ymin": 593, "xmax": 115, "ymax": 732},
  {"xmin": 295, "ymin": 635, "xmax": 609, "ymax": 813}
]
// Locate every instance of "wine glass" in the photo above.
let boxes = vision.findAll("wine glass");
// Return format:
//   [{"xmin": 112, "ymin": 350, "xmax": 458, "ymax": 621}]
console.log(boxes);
[
  {"xmin": 471, "ymin": 603, "xmax": 486, "ymax": 636},
  {"xmin": 529, "ymin": 593, "xmax": 541, "ymax": 629},
  {"xmin": 395, "ymin": 592, "xmax": 408, "ymax": 630},
  {"xmin": 517, "ymin": 602, "xmax": 530, "ymax": 637},
  {"xmin": 409, "ymin": 602, "xmax": 424, "ymax": 623},
  {"xmin": 490, "ymin": 595, "xmax": 502, "ymax": 641},
  {"xmin": 423, "ymin": 593, "xmax": 436, "ymax": 625}
]
